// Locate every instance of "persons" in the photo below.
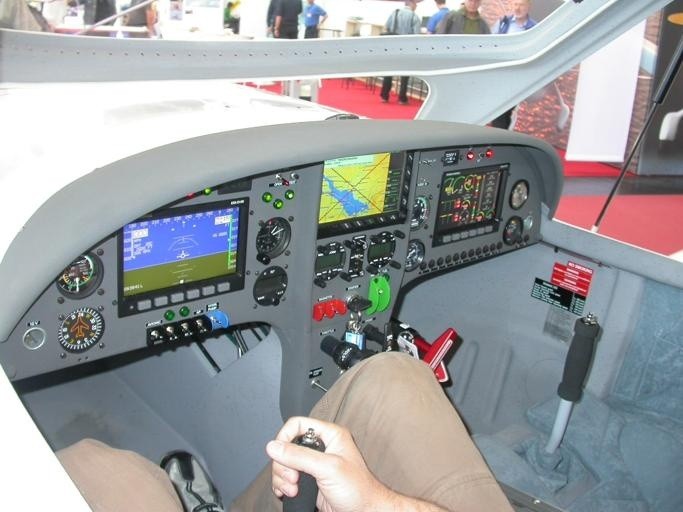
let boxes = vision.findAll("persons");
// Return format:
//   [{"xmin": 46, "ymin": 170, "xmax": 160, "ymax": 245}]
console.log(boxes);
[
  {"xmin": 26, "ymin": 0, "xmax": 183, "ymax": 40},
  {"xmin": 301, "ymin": 1, "xmax": 327, "ymax": 39},
  {"xmin": 426, "ymin": 0, "xmax": 451, "ymax": 34},
  {"xmin": 55, "ymin": 350, "xmax": 515, "ymax": 512},
  {"xmin": 487, "ymin": 1, "xmax": 537, "ymax": 129},
  {"xmin": 436, "ymin": 0, "xmax": 491, "ymax": 34},
  {"xmin": 266, "ymin": 1, "xmax": 302, "ymax": 40},
  {"xmin": 223, "ymin": 2, "xmax": 239, "ymax": 35},
  {"xmin": 379, "ymin": 0, "xmax": 421, "ymax": 106}
]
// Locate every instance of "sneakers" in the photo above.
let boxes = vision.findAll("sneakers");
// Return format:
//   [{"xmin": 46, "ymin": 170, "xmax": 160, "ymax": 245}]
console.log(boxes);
[{"xmin": 160, "ymin": 451, "xmax": 224, "ymax": 512}]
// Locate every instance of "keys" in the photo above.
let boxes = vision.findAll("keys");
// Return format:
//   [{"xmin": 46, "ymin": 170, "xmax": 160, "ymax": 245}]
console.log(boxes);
[{"xmin": 345, "ymin": 296, "xmax": 373, "ymax": 314}]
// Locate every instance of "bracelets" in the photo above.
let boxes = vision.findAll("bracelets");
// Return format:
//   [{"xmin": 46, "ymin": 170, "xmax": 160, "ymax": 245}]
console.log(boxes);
[{"xmin": 273, "ymin": 27, "xmax": 278, "ymax": 30}]
